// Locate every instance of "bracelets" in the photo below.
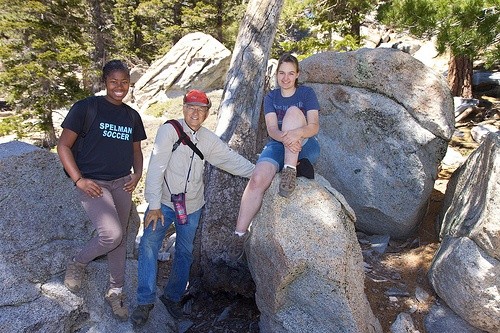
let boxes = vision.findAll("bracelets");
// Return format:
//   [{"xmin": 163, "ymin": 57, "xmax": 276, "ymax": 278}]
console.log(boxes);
[{"xmin": 74, "ymin": 178, "xmax": 81, "ymax": 186}]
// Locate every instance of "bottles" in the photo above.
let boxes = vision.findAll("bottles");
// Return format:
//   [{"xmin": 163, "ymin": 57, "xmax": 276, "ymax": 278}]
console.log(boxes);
[{"xmin": 174, "ymin": 202, "xmax": 187, "ymax": 225}]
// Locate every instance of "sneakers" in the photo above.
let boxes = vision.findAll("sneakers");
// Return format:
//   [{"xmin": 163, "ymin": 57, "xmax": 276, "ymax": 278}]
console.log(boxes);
[
  {"xmin": 227, "ymin": 231, "xmax": 250, "ymax": 260},
  {"xmin": 64, "ymin": 255, "xmax": 88, "ymax": 292},
  {"xmin": 160, "ymin": 294, "xmax": 181, "ymax": 318},
  {"xmin": 279, "ymin": 166, "xmax": 297, "ymax": 196},
  {"xmin": 105, "ymin": 288, "xmax": 129, "ymax": 322},
  {"xmin": 131, "ymin": 304, "xmax": 154, "ymax": 325}
]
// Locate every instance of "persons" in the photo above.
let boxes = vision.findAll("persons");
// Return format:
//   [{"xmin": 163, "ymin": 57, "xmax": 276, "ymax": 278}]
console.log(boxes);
[
  {"xmin": 56, "ymin": 60, "xmax": 147, "ymax": 321},
  {"xmin": 226, "ymin": 54, "xmax": 321, "ymax": 262},
  {"xmin": 131, "ymin": 90, "xmax": 257, "ymax": 329}
]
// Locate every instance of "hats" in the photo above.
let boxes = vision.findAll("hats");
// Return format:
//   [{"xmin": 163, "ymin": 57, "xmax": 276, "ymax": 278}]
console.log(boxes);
[
  {"xmin": 183, "ymin": 90, "xmax": 212, "ymax": 108},
  {"xmin": 296, "ymin": 158, "xmax": 314, "ymax": 179}
]
